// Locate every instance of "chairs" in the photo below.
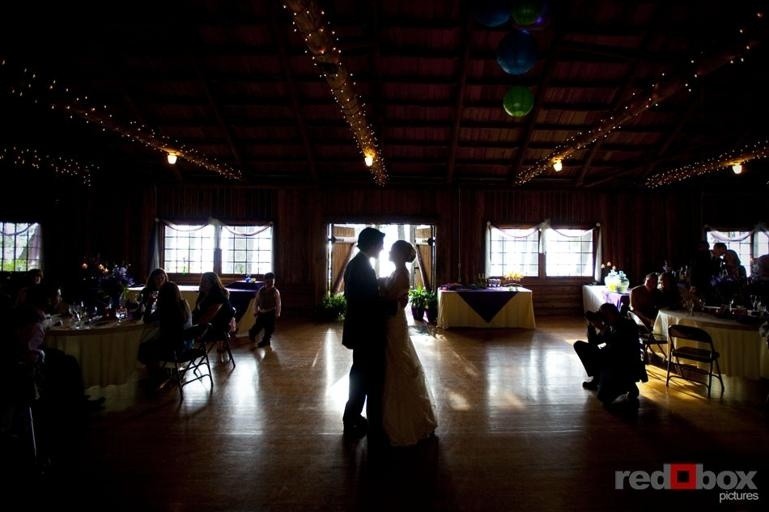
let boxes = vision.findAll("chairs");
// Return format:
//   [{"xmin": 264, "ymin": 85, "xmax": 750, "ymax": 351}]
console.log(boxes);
[
  {"xmin": 0, "ymin": 257, "xmax": 265, "ymax": 458},
  {"xmin": 665, "ymin": 323, "xmax": 726, "ymax": 400},
  {"xmin": 623, "ymin": 305, "xmax": 668, "ymax": 367}
]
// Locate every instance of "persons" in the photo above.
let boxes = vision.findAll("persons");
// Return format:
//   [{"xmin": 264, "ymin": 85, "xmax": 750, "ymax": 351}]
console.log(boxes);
[
  {"xmin": 574, "ymin": 243, "xmax": 768, "ymax": 406},
  {"xmin": 7, "ymin": 269, "xmax": 105, "ymax": 416},
  {"xmin": 248, "ymin": 274, "xmax": 282, "ymax": 347},
  {"xmin": 376, "ymin": 240, "xmax": 438, "ymax": 446},
  {"xmin": 343, "ymin": 227, "xmax": 408, "ymax": 447},
  {"xmin": 143, "ymin": 268, "xmax": 236, "ymax": 392}
]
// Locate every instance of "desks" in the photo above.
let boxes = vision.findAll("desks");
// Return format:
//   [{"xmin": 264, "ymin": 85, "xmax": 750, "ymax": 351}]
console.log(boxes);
[
  {"xmin": 435, "ymin": 283, "xmax": 539, "ymax": 335},
  {"xmin": 579, "ymin": 280, "xmax": 634, "ymax": 321},
  {"xmin": 647, "ymin": 306, "xmax": 768, "ymax": 383}
]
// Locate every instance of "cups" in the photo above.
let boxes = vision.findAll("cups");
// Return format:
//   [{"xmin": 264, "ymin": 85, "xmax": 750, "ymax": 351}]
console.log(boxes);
[
  {"xmin": 487, "ymin": 278, "xmax": 501, "ymax": 287},
  {"xmin": 245, "ymin": 277, "xmax": 256, "ymax": 283}
]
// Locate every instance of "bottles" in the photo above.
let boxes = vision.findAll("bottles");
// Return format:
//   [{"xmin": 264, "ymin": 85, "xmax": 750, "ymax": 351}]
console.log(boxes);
[
  {"xmin": 474, "ymin": 273, "xmax": 485, "ymax": 288},
  {"xmin": 604, "ymin": 268, "xmax": 630, "ymax": 295}
]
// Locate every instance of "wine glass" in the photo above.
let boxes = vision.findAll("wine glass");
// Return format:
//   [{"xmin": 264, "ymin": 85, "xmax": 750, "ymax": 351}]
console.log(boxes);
[
  {"xmin": 115, "ymin": 306, "xmax": 128, "ymax": 321},
  {"xmin": 750, "ymin": 295, "xmax": 761, "ymax": 312},
  {"xmin": 759, "ymin": 304, "xmax": 767, "ymax": 315},
  {"xmin": 681, "ymin": 264, "xmax": 688, "ymax": 279},
  {"xmin": 67, "ymin": 300, "xmax": 89, "ymax": 327}
]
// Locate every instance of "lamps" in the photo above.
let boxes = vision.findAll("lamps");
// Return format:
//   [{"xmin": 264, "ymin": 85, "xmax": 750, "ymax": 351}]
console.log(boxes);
[
  {"xmin": 729, "ymin": 161, "xmax": 744, "ymax": 176},
  {"xmin": 363, "ymin": 152, "xmax": 376, "ymax": 167},
  {"xmin": 165, "ymin": 149, "xmax": 179, "ymax": 165},
  {"xmin": 551, "ymin": 156, "xmax": 566, "ymax": 174}
]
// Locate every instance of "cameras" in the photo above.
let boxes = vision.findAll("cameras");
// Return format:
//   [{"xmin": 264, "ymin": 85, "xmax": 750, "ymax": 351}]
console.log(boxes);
[{"xmin": 585, "ymin": 311, "xmax": 607, "ymax": 327}]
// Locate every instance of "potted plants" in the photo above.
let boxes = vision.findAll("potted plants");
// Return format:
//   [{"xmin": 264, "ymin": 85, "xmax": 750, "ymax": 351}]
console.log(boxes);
[
  {"xmin": 407, "ymin": 280, "xmax": 427, "ymax": 321},
  {"xmin": 422, "ymin": 290, "xmax": 438, "ymax": 323}
]
